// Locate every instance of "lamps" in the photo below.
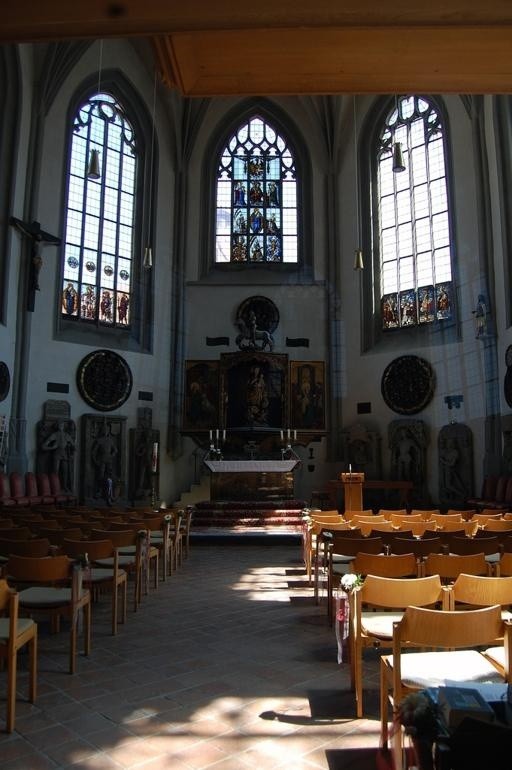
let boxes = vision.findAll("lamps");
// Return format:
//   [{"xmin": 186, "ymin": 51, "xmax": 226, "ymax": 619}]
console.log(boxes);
[
  {"xmin": 144, "ymin": 70, "xmax": 157, "ymax": 267},
  {"xmin": 392, "ymin": 93, "xmax": 406, "ymax": 172},
  {"xmin": 353, "ymin": 95, "xmax": 363, "ymax": 271},
  {"xmin": 87, "ymin": 38, "xmax": 103, "ymax": 179}
]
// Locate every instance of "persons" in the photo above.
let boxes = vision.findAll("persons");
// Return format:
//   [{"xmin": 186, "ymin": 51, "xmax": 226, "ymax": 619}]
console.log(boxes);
[
  {"xmin": 392, "ymin": 428, "xmax": 421, "ymax": 482},
  {"xmin": 135, "ymin": 430, "xmax": 155, "ymax": 495},
  {"xmin": 16, "ymin": 222, "xmax": 60, "ymax": 291},
  {"xmin": 472, "ymin": 294, "xmax": 486, "ymax": 339},
  {"xmin": 42, "ymin": 419, "xmax": 76, "ymax": 494},
  {"xmin": 440, "ymin": 439, "xmax": 467, "ymax": 497},
  {"xmin": 91, "ymin": 425, "xmax": 117, "ymax": 484},
  {"xmin": 249, "ymin": 311, "xmax": 256, "ymax": 345}
]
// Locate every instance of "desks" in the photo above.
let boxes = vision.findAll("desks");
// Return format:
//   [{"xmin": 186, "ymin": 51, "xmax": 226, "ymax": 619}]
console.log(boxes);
[{"xmin": 327, "ymin": 480, "xmax": 413, "ymax": 507}]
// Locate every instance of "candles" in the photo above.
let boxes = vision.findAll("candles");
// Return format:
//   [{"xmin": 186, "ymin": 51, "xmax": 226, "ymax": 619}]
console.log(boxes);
[
  {"xmin": 280, "ymin": 428, "xmax": 297, "ymax": 440},
  {"xmin": 209, "ymin": 429, "xmax": 226, "ymax": 440}
]
